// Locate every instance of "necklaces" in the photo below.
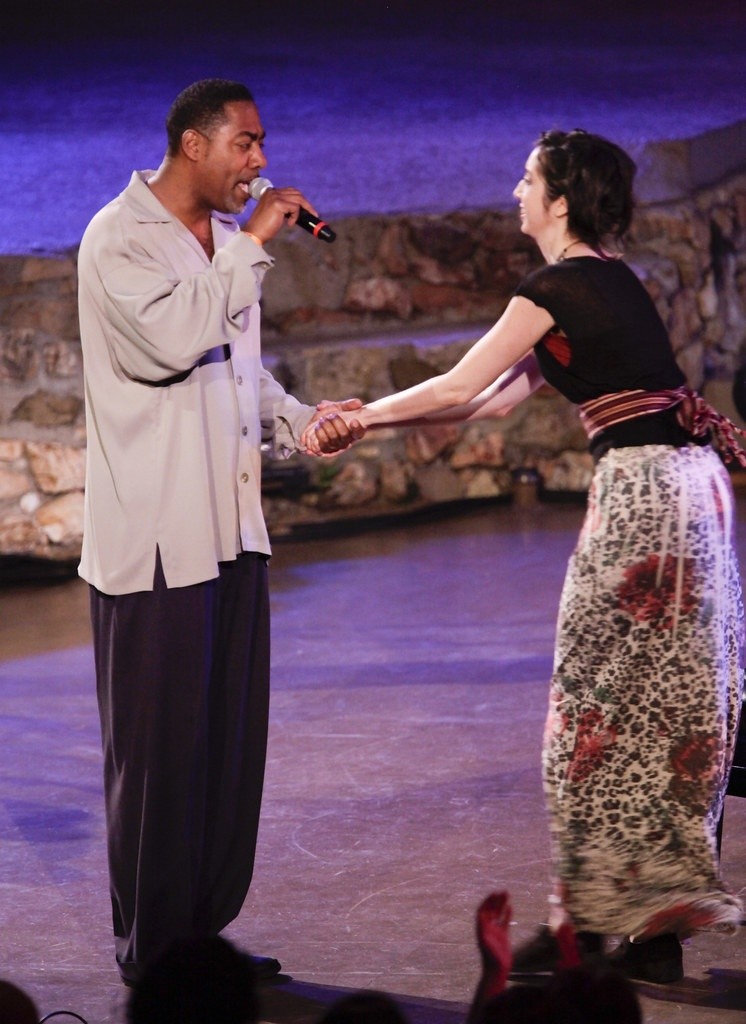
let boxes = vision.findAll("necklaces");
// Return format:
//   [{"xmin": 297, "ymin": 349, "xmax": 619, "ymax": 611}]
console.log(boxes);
[{"xmin": 554, "ymin": 239, "xmax": 588, "ymax": 262}]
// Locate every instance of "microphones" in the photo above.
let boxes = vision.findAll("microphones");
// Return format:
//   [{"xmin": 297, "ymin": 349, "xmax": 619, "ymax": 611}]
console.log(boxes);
[{"xmin": 248, "ymin": 177, "xmax": 337, "ymax": 243}]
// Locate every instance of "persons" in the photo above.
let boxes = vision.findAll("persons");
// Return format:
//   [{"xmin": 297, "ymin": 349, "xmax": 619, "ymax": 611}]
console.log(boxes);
[
  {"xmin": 299, "ymin": 118, "xmax": 746, "ymax": 992},
  {"xmin": 68, "ymin": 74, "xmax": 369, "ymax": 1024}
]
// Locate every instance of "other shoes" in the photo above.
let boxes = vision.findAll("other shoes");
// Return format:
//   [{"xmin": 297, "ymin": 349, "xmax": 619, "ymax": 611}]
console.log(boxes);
[
  {"xmin": 237, "ymin": 950, "xmax": 283, "ymax": 978},
  {"xmin": 502, "ymin": 916, "xmax": 690, "ymax": 994}
]
